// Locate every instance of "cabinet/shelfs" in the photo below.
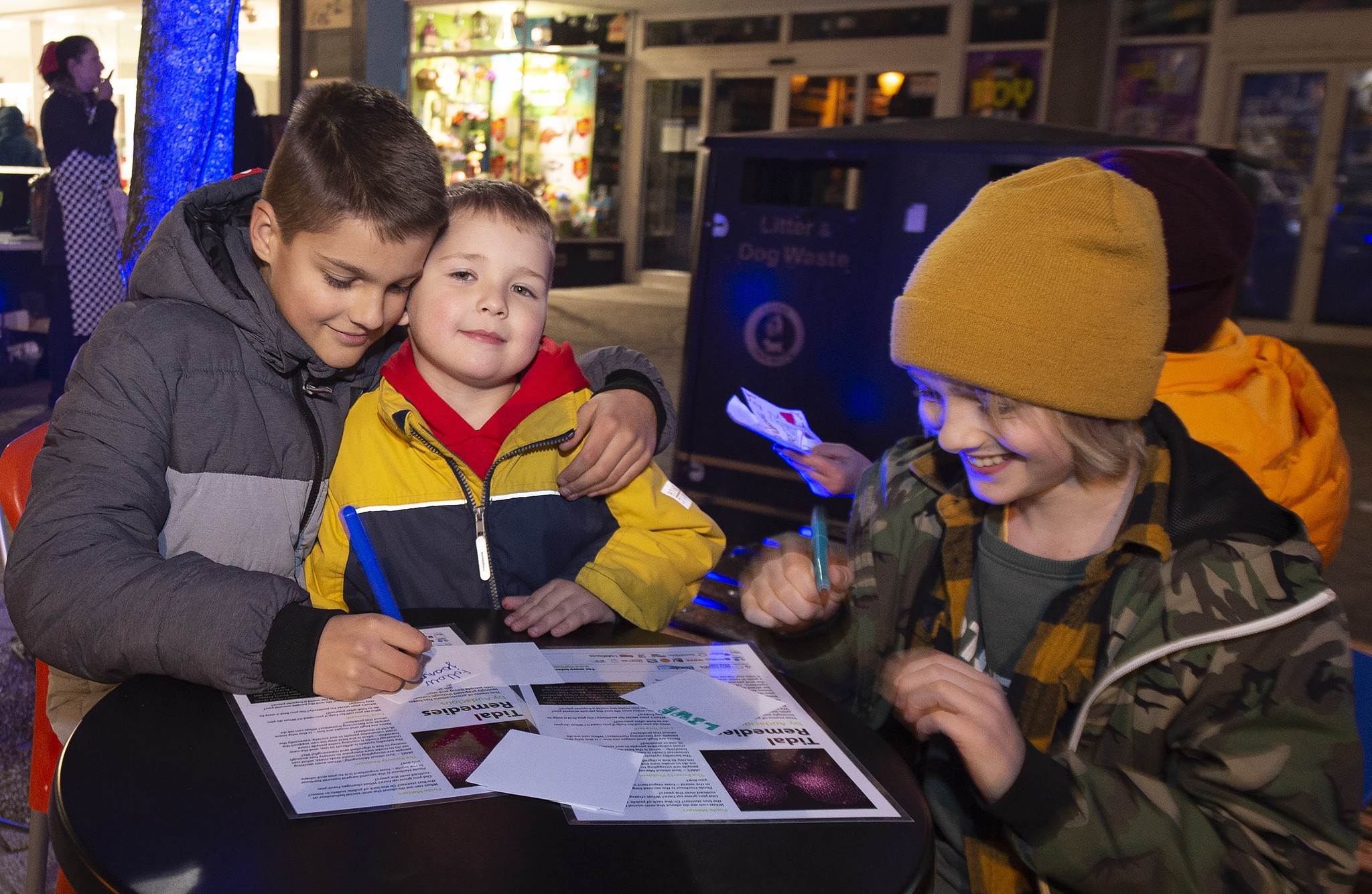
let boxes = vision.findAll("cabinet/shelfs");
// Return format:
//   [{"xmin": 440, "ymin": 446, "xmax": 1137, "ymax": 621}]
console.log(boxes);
[{"xmin": 0, "ymin": 165, "xmax": 50, "ymax": 378}]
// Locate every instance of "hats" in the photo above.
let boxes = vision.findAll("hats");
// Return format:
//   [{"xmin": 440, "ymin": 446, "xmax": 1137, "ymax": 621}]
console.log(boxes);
[
  {"xmin": 890, "ymin": 157, "xmax": 1169, "ymax": 421},
  {"xmin": 1087, "ymin": 148, "xmax": 1251, "ymax": 350}
]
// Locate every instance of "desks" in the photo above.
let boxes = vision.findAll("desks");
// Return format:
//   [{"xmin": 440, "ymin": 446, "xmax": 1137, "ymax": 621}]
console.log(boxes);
[{"xmin": 48, "ymin": 607, "xmax": 936, "ymax": 894}]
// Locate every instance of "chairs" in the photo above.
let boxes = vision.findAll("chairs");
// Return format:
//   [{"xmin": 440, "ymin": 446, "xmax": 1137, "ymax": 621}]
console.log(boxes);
[{"xmin": 0, "ymin": 418, "xmax": 75, "ymax": 894}]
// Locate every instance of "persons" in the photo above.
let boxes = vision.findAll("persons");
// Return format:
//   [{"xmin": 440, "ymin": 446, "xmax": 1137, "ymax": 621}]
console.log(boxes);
[
  {"xmin": 0, "ymin": 35, "xmax": 129, "ymax": 415},
  {"xmin": 233, "ymin": 71, "xmax": 266, "ymax": 175},
  {"xmin": 303, "ymin": 180, "xmax": 725, "ymax": 638},
  {"xmin": 4, "ymin": 81, "xmax": 678, "ymax": 745},
  {"xmin": 738, "ymin": 146, "xmax": 1361, "ymax": 894}
]
